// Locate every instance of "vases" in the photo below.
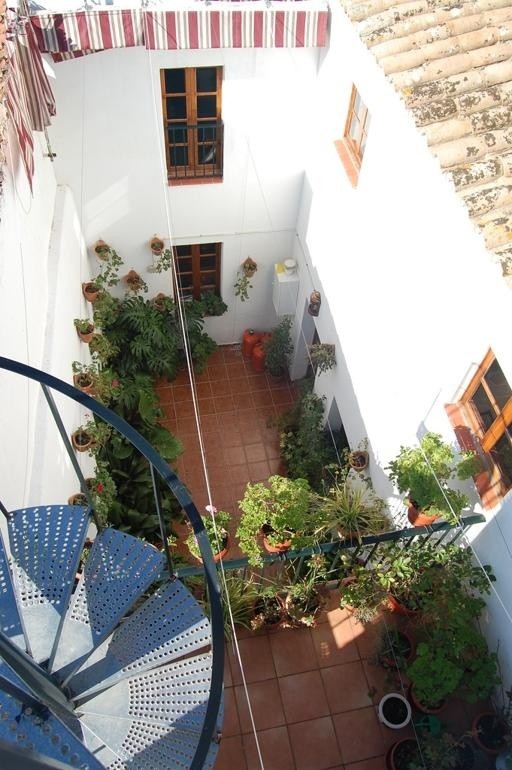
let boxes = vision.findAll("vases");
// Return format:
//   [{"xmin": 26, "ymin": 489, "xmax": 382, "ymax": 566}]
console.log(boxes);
[
  {"xmin": 184, "ymin": 535, "xmax": 232, "ymax": 565},
  {"xmin": 262, "ymin": 533, "xmax": 292, "ymax": 553}
]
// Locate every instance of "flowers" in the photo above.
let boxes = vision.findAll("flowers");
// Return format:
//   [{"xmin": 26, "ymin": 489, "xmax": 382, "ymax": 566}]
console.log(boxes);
[
  {"xmin": 233, "ymin": 474, "xmax": 326, "ymax": 569},
  {"xmin": 183, "ymin": 503, "xmax": 233, "ymax": 558},
  {"xmin": 249, "ymin": 554, "xmax": 332, "ymax": 633}
]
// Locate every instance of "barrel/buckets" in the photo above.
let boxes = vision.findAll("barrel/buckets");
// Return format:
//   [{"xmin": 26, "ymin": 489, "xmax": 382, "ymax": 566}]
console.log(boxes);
[
  {"xmin": 253, "ymin": 342, "xmax": 266, "ymax": 372},
  {"xmin": 241, "ymin": 327, "xmax": 259, "ymax": 357}
]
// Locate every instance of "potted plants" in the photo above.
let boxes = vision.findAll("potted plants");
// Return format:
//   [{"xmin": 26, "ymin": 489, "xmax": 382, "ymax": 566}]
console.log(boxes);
[
  {"xmin": 310, "ymin": 431, "xmax": 511, "ymax": 770},
  {"xmin": 262, "ymin": 315, "xmax": 296, "ymax": 383},
  {"xmin": 93, "ymin": 233, "xmax": 172, "ymax": 294},
  {"xmin": 232, "ymin": 256, "xmax": 258, "ymax": 302},
  {"xmin": 68, "ymin": 281, "xmax": 103, "ymax": 522}
]
